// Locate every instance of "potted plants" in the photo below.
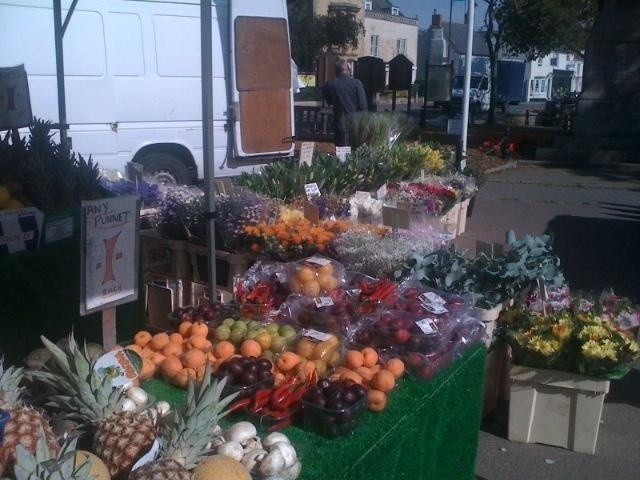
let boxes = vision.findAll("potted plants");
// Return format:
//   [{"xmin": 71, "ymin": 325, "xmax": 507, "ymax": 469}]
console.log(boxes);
[{"xmin": 460, "ymin": 164, "xmax": 486, "ymax": 217}]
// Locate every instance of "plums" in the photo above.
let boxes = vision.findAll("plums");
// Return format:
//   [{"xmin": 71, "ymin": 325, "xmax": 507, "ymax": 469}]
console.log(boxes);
[
  {"xmin": 302, "ymin": 378, "xmax": 368, "ymax": 437},
  {"xmin": 125, "ymin": 264, "xmax": 480, "ymax": 412}
]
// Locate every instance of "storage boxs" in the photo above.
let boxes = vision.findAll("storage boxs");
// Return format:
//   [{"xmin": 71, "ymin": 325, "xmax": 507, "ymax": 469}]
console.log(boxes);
[
  {"xmin": 140, "ymin": 231, "xmax": 254, "ymax": 331},
  {"xmin": 482, "ymin": 344, "xmax": 511, "ymax": 423},
  {"xmin": 0, "ymin": 208, "xmax": 44, "ymax": 253},
  {"xmin": 471, "ymin": 299, "xmax": 514, "ymax": 350},
  {"xmin": 507, "ymin": 360, "xmax": 610, "ymax": 455}
]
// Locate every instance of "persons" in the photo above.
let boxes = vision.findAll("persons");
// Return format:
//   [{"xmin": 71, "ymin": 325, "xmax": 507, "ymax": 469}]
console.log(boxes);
[{"xmin": 324, "ymin": 60, "xmax": 368, "ymax": 148}]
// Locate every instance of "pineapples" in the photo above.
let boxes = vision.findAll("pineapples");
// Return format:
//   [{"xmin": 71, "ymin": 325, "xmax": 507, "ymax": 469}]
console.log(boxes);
[{"xmin": 0, "ymin": 325, "xmax": 241, "ymax": 480}]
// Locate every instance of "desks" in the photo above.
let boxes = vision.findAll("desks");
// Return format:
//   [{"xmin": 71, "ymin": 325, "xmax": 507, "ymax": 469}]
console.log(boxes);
[
  {"xmin": 0, "ymin": 233, "xmax": 147, "ymax": 372},
  {"xmin": 115, "ymin": 336, "xmax": 486, "ymax": 480}
]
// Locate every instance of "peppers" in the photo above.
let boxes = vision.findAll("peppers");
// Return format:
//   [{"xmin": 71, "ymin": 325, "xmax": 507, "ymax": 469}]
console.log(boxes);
[{"xmin": 229, "ymin": 368, "xmax": 317, "ymax": 432}]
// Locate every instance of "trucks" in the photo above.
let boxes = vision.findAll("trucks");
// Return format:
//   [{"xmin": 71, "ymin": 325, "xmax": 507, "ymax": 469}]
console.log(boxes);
[
  {"xmin": 0, "ymin": 1, "xmax": 295, "ymax": 186},
  {"xmin": 451, "ymin": 58, "xmax": 526, "ymax": 112}
]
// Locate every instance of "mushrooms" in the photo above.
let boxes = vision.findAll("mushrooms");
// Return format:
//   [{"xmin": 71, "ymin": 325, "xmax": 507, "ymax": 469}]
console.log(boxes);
[{"xmin": 120, "ymin": 386, "xmax": 301, "ymax": 480}]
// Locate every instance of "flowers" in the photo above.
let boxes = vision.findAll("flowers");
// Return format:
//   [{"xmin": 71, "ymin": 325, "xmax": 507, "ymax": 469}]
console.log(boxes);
[
  {"xmin": 499, "ymin": 277, "xmax": 639, "ymax": 380},
  {"xmin": 109, "ymin": 177, "xmax": 478, "ymax": 270}
]
